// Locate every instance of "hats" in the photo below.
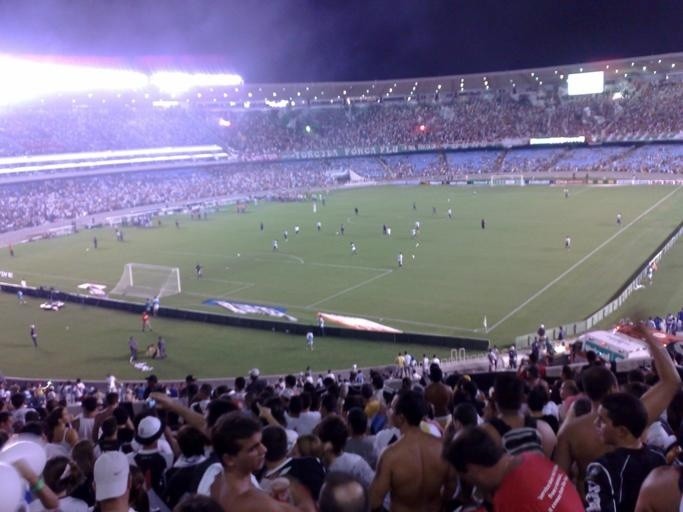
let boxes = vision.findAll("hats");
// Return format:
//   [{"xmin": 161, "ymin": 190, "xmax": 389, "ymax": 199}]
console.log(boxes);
[
  {"xmin": 0, "ymin": 463, "xmax": 22, "ymax": 512},
  {"xmin": 144, "ymin": 375, "xmax": 157, "ymax": 383},
  {"xmin": 135, "ymin": 416, "xmax": 166, "ymax": 445},
  {"xmin": 248, "ymin": 368, "xmax": 259, "ymax": 376},
  {"xmin": 92, "ymin": 450, "xmax": 130, "ymax": 501},
  {"xmin": 186, "ymin": 375, "xmax": 196, "ymax": 381}
]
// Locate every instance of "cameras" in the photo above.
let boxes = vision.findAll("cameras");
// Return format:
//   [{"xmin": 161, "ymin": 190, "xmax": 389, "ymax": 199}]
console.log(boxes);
[{"xmin": 142, "ymin": 397, "xmax": 157, "ymax": 410}]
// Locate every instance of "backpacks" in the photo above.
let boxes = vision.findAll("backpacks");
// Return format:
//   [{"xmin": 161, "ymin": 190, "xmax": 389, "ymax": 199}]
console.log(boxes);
[{"xmin": 489, "ymin": 416, "xmax": 543, "ymax": 456}]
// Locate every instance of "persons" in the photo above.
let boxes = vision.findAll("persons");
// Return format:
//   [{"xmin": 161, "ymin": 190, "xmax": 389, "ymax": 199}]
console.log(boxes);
[
  {"xmin": 397, "ymin": 253, "xmax": 403, "ymax": 266},
  {"xmin": 0, "ymin": 312, "xmax": 681, "ymax": 512},
  {"xmin": 564, "ymin": 238, "xmax": 575, "ymax": 251},
  {"xmin": 91, "ymin": 236, "xmax": 97, "ymax": 247},
  {"xmin": 19, "ymin": 279, "xmax": 26, "ymax": 301},
  {"xmin": 349, "ymin": 241, "xmax": 359, "ymax": 258},
  {"xmin": 194, "ymin": 263, "xmax": 202, "ymax": 276},
  {"xmin": 273, "ymin": 239, "xmax": 276, "ymax": 251},
  {"xmin": 8, "ymin": 241, "xmax": 15, "ymax": 257},
  {"xmin": 1, "ymin": 73, "xmax": 683, "ymax": 239},
  {"xmin": 138, "ymin": 294, "xmax": 162, "ymax": 313}
]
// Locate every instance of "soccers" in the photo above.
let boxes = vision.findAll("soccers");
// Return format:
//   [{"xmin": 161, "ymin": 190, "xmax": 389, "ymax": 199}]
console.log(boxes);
[
  {"xmin": 237, "ymin": 253, "xmax": 240, "ymax": 256},
  {"xmin": 86, "ymin": 248, "xmax": 89, "ymax": 251}
]
